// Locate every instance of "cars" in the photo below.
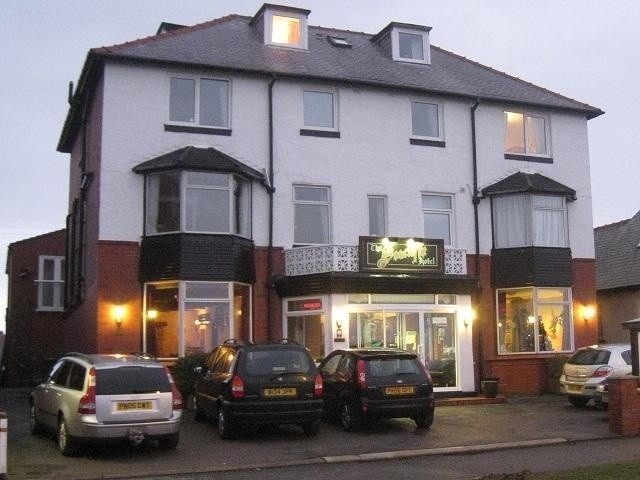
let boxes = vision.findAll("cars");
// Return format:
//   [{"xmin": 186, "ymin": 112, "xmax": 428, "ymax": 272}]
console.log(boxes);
[{"xmin": 560, "ymin": 343, "xmax": 632, "ymax": 410}]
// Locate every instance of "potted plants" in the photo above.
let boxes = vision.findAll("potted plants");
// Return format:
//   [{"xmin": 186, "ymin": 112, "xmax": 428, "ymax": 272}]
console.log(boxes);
[
  {"xmin": 171, "ymin": 353, "xmax": 208, "ymax": 411},
  {"xmin": 481, "ymin": 378, "xmax": 501, "ymax": 398}
]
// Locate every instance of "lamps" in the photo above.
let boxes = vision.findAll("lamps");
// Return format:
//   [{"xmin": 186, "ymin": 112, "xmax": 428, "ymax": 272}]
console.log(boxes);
[
  {"xmin": 336, "ymin": 319, "xmax": 342, "ymax": 327},
  {"xmin": 18, "ymin": 267, "xmax": 29, "ymax": 278},
  {"xmin": 464, "ymin": 319, "xmax": 469, "ymax": 327},
  {"xmin": 147, "ymin": 308, "xmax": 158, "ymax": 322},
  {"xmin": 114, "ymin": 309, "xmax": 122, "ymax": 328}
]
